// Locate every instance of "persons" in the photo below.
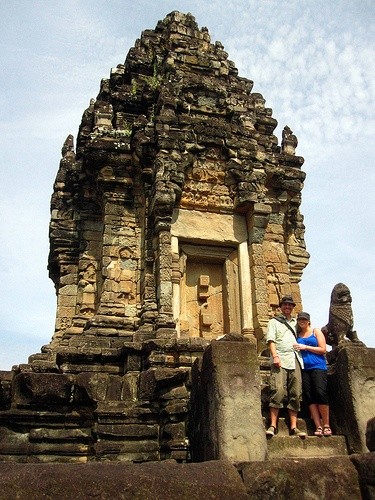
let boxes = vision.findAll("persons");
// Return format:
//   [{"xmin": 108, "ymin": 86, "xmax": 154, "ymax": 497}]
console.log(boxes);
[
  {"xmin": 294, "ymin": 312, "xmax": 332, "ymax": 436},
  {"xmin": 265, "ymin": 296, "xmax": 308, "ymax": 437}
]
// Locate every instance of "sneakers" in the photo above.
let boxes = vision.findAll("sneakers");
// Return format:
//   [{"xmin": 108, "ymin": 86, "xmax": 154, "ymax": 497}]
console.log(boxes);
[
  {"xmin": 289, "ymin": 428, "xmax": 306, "ymax": 436},
  {"xmin": 266, "ymin": 426, "xmax": 278, "ymax": 436}
]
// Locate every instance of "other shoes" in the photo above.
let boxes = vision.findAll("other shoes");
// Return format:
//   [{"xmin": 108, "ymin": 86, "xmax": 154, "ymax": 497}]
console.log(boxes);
[
  {"xmin": 323, "ymin": 427, "xmax": 332, "ymax": 436},
  {"xmin": 314, "ymin": 428, "xmax": 323, "ymax": 436}
]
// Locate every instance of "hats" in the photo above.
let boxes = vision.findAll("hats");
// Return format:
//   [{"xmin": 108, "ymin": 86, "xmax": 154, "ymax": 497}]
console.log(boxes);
[
  {"xmin": 297, "ymin": 312, "xmax": 310, "ymax": 320},
  {"xmin": 279, "ymin": 296, "xmax": 296, "ymax": 307}
]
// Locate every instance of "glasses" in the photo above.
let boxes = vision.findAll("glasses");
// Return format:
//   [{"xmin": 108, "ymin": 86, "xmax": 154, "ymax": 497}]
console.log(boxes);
[
  {"xmin": 298, "ymin": 318, "xmax": 307, "ymax": 321},
  {"xmin": 282, "ymin": 297, "xmax": 293, "ymax": 301}
]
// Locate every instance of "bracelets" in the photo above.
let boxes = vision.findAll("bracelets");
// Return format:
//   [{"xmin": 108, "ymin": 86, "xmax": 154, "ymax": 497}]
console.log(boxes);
[{"xmin": 273, "ymin": 356, "xmax": 279, "ymax": 358}]
